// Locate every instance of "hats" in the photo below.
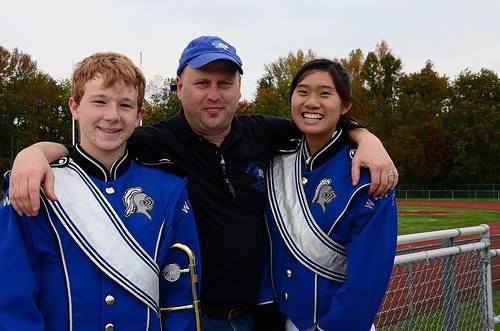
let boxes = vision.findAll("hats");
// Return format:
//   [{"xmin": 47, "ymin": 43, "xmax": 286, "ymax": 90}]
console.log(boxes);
[{"xmin": 177, "ymin": 36, "xmax": 244, "ymax": 76}]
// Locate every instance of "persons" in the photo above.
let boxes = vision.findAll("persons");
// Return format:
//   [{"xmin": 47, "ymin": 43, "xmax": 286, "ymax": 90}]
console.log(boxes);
[
  {"xmin": 0, "ymin": 51, "xmax": 201, "ymax": 331},
  {"xmin": 256, "ymin": 59, "xmax": 398, "ymax": 331},
  {"xmin": 9, "ymin": 36, "xmax": 398, "ymax": 331}
]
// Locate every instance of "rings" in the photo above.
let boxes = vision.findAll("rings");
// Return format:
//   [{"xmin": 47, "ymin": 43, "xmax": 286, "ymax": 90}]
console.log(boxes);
[{"xmin": 388, "ymin": 174, "xmax": 394, "ymax": 176}]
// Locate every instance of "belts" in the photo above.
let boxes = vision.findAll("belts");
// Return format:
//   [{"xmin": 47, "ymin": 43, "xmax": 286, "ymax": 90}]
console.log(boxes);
[{"xmin": 201, "ymin": 302, "xmax": 256, "ymax": 320}]
